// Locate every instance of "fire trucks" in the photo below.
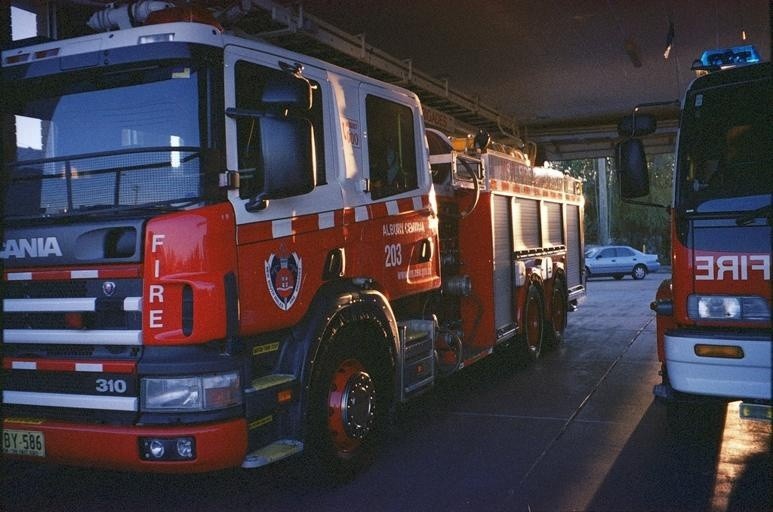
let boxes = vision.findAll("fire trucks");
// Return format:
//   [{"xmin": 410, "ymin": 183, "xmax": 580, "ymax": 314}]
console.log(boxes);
[
  {"xmin": 1, "ymin": 0, "xmax": 586, "ymax": 487},
  {"xmin": 608, "ymin": 42, "xmax": 771, "ymax": 471}
]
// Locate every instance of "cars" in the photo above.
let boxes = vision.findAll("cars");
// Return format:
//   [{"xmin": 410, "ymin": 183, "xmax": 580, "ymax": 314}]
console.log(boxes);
[{"xmin": 584, "ymin": 244, "xmax": 660, "ymax": 282}]
